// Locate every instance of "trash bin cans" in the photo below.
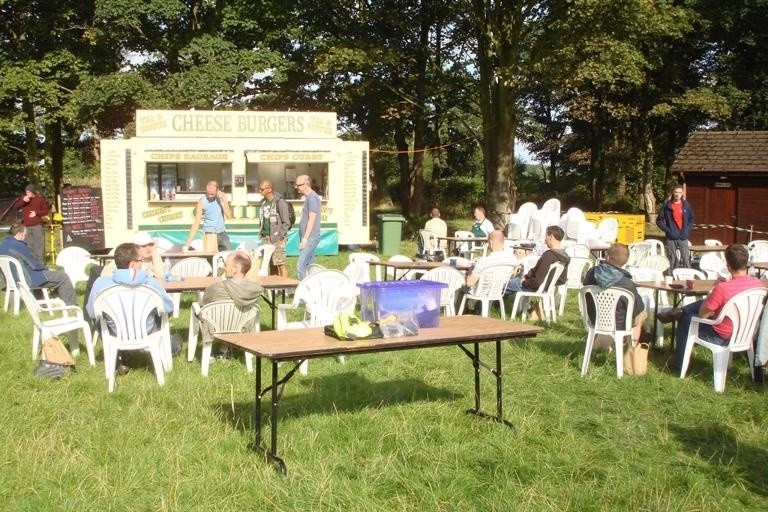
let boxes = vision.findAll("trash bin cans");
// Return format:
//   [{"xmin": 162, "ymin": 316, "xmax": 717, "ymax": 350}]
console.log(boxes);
[{"xmin": 377, "ymin": 213, "xmax": 406, "ymax": 257}]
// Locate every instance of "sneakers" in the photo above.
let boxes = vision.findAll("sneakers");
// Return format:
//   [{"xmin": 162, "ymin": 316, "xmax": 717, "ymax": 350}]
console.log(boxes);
[
  {"xmin": 657, "ymin": 309, "xmax": 683, "ymax": 323},
  {"xmin": 219, "ymin": 343, "xmax": 229, "ymax": 354},
  {"xmin": 171, "ymin": 333, "xmax": 183, "ymax": 356},
  {"xmin": 118, "ymin": 351, "xmax": 131, "ymax": 374}
]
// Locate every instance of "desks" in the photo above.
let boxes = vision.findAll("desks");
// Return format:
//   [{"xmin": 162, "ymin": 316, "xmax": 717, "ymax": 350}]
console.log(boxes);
[{"xmin": 211, "ymin": 314, "xmax": 544, "ymax": 473}]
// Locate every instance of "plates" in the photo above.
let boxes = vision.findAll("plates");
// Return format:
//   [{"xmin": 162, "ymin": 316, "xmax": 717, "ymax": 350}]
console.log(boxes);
[{"xmin": 669, "ymin": 284, "xmax": 684, "ymax": 289}]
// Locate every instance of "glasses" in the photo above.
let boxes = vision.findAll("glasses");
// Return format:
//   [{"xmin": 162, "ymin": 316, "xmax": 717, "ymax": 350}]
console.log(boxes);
[
  {"xmin": 295, "ymin": 183, "xmax": 306, "ymax": 187},
  {"xmin": 258, "ymin": 187, "xmax": 271, "ymax": 191},
  {"xmin": 135, "ymin": 257, "xmax": 145, "ymax": 263}
]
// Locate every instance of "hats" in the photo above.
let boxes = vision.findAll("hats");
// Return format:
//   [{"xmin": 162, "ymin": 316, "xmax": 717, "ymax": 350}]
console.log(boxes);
[
  {"xmin": 134, "ymin": 231, "xmax": 152, "ymax": 245},
  {"xmin": 26, "ymin": 184, "xmax": 37, "ymax": 192}
]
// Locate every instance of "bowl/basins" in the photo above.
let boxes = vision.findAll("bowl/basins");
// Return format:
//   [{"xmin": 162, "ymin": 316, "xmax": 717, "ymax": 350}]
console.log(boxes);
[{"xmin": 520, "ymin": 243, "xmax": 536, "ymax": 248}]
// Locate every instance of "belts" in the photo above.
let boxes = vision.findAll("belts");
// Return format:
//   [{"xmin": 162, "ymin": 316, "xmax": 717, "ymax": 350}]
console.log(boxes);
[{"xmin": 205, "ymin": 231, "xmax": 224, "ymax": 234}]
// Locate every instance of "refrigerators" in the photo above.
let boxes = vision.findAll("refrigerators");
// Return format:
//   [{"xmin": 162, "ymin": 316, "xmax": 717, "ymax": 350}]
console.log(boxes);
[{"xmin": 146, "ymin": 163, "xmax": 178, "ymax": 199}]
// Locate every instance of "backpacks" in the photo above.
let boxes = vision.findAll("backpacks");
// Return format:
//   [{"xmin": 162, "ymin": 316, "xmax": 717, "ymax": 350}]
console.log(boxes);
[{"xmin": 259, "ymin": 197, "xmax": 296, "ymax": 230}]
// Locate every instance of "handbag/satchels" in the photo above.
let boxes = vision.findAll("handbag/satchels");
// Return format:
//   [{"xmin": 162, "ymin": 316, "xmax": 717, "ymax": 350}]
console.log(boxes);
[
  {"xmin": 624, "ymin": 339, "xmax": 650, "ymax": 375},
  {"xmin": 325, "ymin": 324, "xmax": 383, "ymax": 340},
  {"xmin": 526, "ymin": 298, "xmax": 546, "ymax": 321}
]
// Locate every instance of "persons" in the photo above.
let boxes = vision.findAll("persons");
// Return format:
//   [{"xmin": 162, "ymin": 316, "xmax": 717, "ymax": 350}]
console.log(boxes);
[
  {"xmin": 582, "ymin": 242, "xmax": 648, "ymax": 351},
  {"xmin": 417, "ymin": 208, "xmax": 450, "ymax": 256},
  {"xmin": 469, "ymin": 206, "xmax": 495, "ymax": 245},
  {"xmin": 98, "ymin": 228, "xmax": 169, "ymax": 281},
  {"xmin": 496, "ymin": 225, "xmax": 572, "ymax": 308},
  {"xmin": 654, "ymin": 182, "xmax": 697, "ymax": 274},
  {"xmin": 198, "ymin": 252, "xmax": 266, "ymax": 357},
  {"xmin": 13, "ymin": 184, "xmax": 51, "ymax": 268},
  {"xmin": 84, "ymin": 241, "xmax": 174, "ymax": 376},
  {"xmin": 657, "ymin": 241, "xmax": 767, "ymax": 374},
  {"xmin": 255, "ymin": 178, "xmax": 297, "ymax": 298},
  {"xmin": 0, "ymin": 224, "xmax": 81, "ymax": 308},
  {"xmin": 181, "ymin": 178, "xmax": 236, "ymax": 273},
  {"xmin": 454, "ymin": 229, "xmax": 519, "ymax": 314},
  {"xmin": 292, "ymin": 171, "xmax": 325, "ymax": 278}
]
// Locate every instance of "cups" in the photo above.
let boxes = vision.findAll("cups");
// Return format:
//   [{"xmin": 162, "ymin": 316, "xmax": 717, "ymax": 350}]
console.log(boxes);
[
  {"xmin": 686, "ymin": 279, "xmax": 695, "ymax": 289},
  {"xmin": 664, "ymin": 276, "xmax": 673, "ymax": 284},
  {"xmin": 182, "ymin": 246, "xmax": 189, "ymax": 253},
  {"xmin": 449, "ymin": 258, "xmax": 457, "ymax": 266},
  {"xmin": 177, "ymin": 268, "xmax": 185, "ymax": 281}
]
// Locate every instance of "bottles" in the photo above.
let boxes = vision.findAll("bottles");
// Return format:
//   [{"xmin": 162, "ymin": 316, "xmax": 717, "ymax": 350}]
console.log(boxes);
[
  {"xmin": 148, "ymin": 166, "xmax": 160, "ymax": 199},
  {"xmin": 161, "ymin": 167, "xmax": 176, "ymax": 199},
  {"xmin": 162, "ymin": 257, "xmax": 173, "ymax": 281}
]
